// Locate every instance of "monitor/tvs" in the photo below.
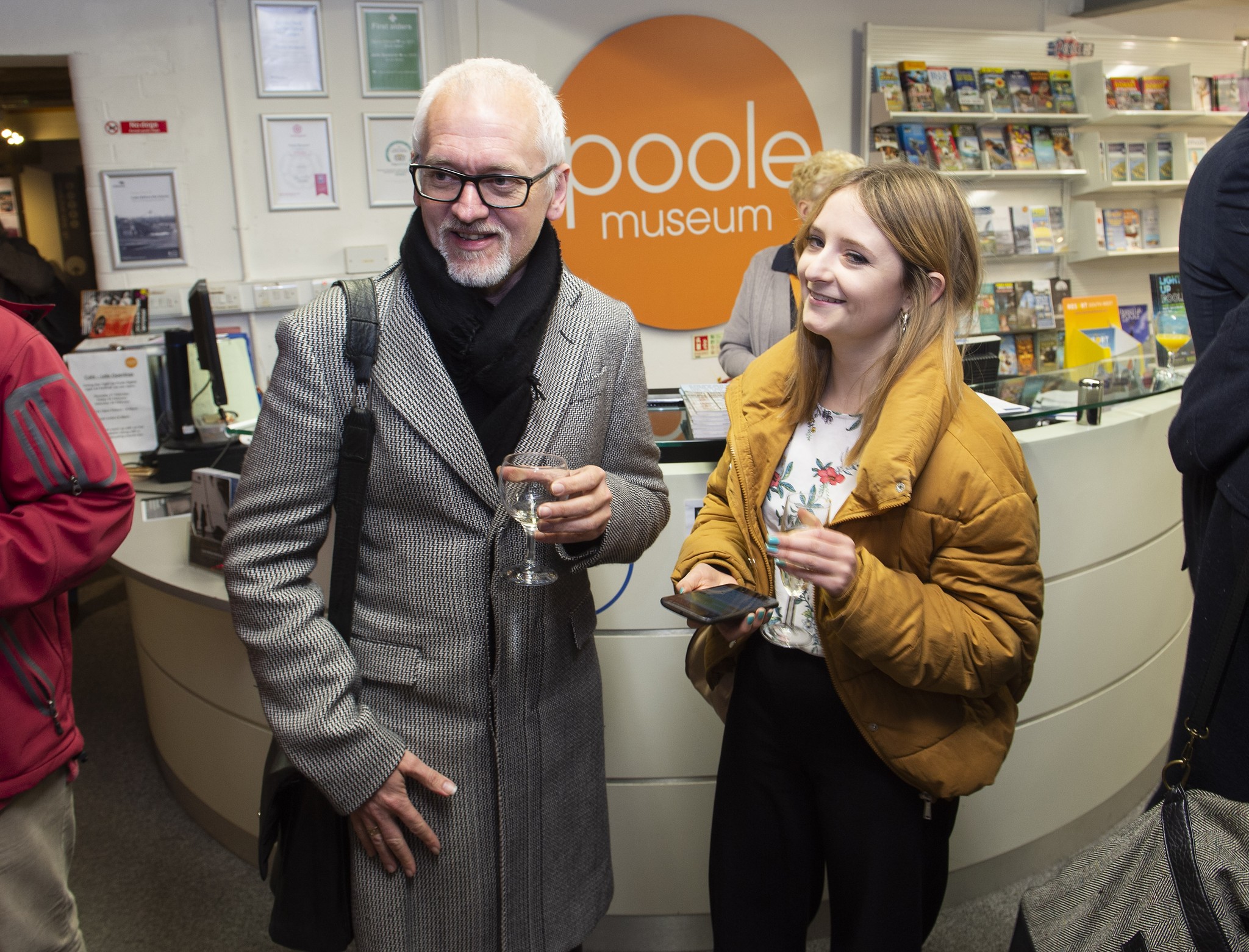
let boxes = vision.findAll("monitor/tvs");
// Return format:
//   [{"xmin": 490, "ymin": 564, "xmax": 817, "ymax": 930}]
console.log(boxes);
[{"xmin": 161, "ymin": 278, "xmax": 241, "ymax": 452}]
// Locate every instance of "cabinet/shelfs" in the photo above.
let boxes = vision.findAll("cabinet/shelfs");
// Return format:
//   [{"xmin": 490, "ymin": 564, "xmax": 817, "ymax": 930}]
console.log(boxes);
[{"xmin": 861, "ymin": 21, "xmax": 1249, "ymax": 407}]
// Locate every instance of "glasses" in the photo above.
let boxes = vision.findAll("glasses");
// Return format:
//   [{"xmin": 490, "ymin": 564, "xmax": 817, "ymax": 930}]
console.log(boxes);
[{"xmin": 409, "ymin": 154, "xmax": 556, "ymax": 209}]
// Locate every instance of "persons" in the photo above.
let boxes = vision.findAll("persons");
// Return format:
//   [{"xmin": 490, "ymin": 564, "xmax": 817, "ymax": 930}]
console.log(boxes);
[
  {"xmin": 217, "ymin": 56, "xmax": 671, "ymax": 952},
  {"xmin": 0, "ymin": 300, "xmax": 134, "ymax": 951},
  {"xmin": 718, "ymin": 150, "xmax": 866, "ymax": 377},
  {"xmin": 1145, "ymin": 120, "xmax": 1249, "ymax": 841},
  {"xmin": 669, "ymin": 167, "xmax": 1044, "ymax": 952}
]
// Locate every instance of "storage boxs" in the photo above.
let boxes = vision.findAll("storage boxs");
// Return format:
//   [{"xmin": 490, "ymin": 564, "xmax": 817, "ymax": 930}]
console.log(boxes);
[{"xmin": 1060, "ymin": 294, "xmax": 1143, "ymax": 381}]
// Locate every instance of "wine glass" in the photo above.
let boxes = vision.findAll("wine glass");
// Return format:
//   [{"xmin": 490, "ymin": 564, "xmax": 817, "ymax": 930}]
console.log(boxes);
[
  {"xmin": 499, "ymin": 452, "xmax": 570, "ymax": 587},
  {"xmin": 1150, "ymin": 310, "xmax": 1192, "ymax": 381},
  {"xmin": 759, "ymin": 493, "xmax": 832, "ymax": 649}
]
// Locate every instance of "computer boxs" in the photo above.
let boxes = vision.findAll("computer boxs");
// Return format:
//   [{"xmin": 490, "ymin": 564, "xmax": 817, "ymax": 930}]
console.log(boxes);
[{"xmin": 156, "ymin": 441, "xmax": 249, "ymax": 483}]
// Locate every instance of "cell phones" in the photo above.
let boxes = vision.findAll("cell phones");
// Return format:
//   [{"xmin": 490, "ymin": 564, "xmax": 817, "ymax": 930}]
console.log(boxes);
[{"xmin": 661, "ymin": 583, "xmax": 780, "ymax": 625}]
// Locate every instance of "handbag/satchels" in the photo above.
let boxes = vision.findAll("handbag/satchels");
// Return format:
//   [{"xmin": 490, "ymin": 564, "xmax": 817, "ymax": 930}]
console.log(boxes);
[
  {"xmin": 1009, "ymin": 790, "xmax": 1249, "ymax": 952},
  {"xmin": 259, "ymin": 735, "xmax": 355, "ymax": 951}
]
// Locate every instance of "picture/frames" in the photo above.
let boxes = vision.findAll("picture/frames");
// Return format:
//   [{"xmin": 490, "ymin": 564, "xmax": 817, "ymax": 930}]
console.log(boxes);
[{"xmin": 100, "ymin": 168, "xmax": 190, "ymax": 270}]
